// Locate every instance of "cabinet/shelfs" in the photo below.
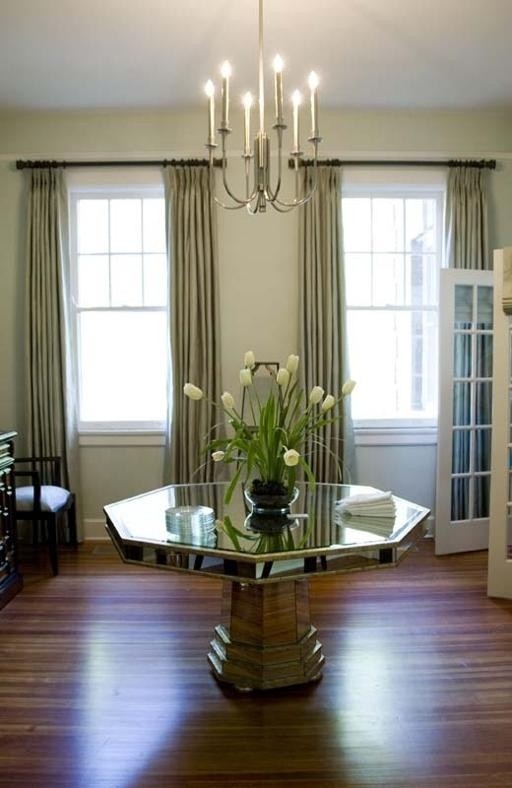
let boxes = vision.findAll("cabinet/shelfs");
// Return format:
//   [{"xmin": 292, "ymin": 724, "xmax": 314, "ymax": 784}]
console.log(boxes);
[{"xmin": 0, "ymin": 430, "xmax": 23, "ymax": 609}]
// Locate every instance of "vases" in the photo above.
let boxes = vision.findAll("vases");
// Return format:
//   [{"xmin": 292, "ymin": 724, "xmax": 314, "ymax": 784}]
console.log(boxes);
[
  {"xmin": 244, "ymin": 505, "xmax": 297, "ymax": 533},
  {"xmin": 243, "ymin": 479, "xmax": 300, "ymax": 515}
]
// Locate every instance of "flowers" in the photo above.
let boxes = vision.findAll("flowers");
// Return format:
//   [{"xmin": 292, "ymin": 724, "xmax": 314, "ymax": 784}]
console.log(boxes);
[
  {"xmin": 213, "ymin": 515, "xmax": 315, "ymax": 552},
  {"xmin": 183, "ymin": 351, "xmax": 356, "ymax": 504}
]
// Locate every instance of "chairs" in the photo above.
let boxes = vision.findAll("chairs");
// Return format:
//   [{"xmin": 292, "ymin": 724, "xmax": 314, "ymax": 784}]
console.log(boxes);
[{"xmin": 11, "ymin": 454, "xmax": 78, "ymax": 575}]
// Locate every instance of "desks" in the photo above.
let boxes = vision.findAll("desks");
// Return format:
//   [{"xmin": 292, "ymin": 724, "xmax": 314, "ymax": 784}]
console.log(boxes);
[{"xmin": 103, "ymin": 483, "xmax": 431, "ymax": 693}]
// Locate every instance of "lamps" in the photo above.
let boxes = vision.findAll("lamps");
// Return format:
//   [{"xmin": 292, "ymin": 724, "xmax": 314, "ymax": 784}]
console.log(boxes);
[{"xmin": 204, "ymin": 0, "xmax": 323, "ymax": 213}]
